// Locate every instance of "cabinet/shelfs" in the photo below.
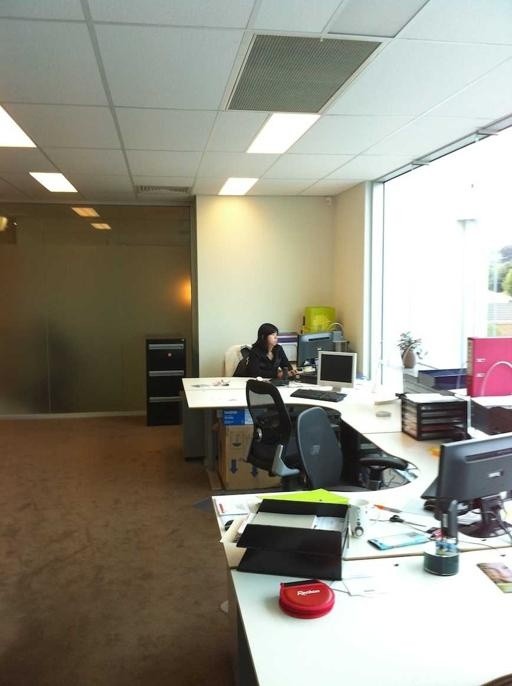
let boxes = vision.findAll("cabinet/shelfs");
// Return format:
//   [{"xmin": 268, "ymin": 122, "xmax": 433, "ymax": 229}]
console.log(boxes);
[{"xmin": 145, "ymin": 338, "xmax": 186, "ymax": 426}]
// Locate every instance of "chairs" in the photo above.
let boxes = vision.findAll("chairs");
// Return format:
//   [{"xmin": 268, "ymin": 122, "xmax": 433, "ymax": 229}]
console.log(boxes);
[{"xmin": 225, "ymin": 344, "xmax": 252, "ymax": 377}]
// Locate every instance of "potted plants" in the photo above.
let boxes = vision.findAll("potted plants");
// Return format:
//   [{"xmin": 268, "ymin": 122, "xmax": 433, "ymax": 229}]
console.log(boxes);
[{"xmin": 396, "ymin": 332, "xmax": 428, "ymax": 367}]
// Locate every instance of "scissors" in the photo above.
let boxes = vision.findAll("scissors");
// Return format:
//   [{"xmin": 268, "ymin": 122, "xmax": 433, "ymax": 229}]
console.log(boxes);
[{"xmin": 391, "ymin": 515, "xmax": 428, "ymax": 527}]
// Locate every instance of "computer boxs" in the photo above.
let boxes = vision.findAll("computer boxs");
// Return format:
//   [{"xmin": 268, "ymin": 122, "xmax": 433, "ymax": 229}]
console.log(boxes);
[{"xmin": 300, "ymin": 371, "xmax": 316, "ymax": 384}]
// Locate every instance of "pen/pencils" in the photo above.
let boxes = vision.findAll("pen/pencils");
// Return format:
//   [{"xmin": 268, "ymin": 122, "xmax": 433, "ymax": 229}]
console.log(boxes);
[{"xmin": 375, "ymin": 504, "xmax": 403, "ymax": 514}]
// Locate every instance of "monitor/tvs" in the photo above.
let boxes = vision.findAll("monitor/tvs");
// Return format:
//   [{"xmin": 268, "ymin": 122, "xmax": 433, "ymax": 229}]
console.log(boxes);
[
  {"xmin": 437, "ymin": 431, "xmax": 511, "ymax": 538},
  {"xmin": 297, "ymin": 332, "xmax": 332, "ymax": 373},
  {"xmin": 316, "ymin": 349, "xmax": 357, "ymax": 391}
]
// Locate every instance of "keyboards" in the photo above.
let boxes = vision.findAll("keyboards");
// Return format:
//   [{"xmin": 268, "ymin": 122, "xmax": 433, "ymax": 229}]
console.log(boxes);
[
  {"xmin": 290, "ymin": 388, "xmax": 347, "ymax": 403},
  {"xmin": 421, "ymin": 476, "xmax": 440, "ymax": 497}
]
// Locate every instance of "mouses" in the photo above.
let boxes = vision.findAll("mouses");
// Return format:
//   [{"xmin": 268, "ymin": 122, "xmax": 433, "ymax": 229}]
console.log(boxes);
[{"xmin": 424, "ymin": 497, "xmax": 436, "ymax": 511}]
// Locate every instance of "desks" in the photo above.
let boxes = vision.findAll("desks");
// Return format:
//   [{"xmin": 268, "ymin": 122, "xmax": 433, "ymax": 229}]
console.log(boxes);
[{"xmin": 182, "ymin": 377, "xmax": 512, "ymax": 686}]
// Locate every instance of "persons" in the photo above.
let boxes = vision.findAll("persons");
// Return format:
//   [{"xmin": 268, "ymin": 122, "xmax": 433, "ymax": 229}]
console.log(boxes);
[
  {"xmin": 244, "ymin": 322, "xmax": 301, "ymax": 377},
  {"xmin": 479, "ymin": 561, "xmax": 512, "ymax": 584}
]
